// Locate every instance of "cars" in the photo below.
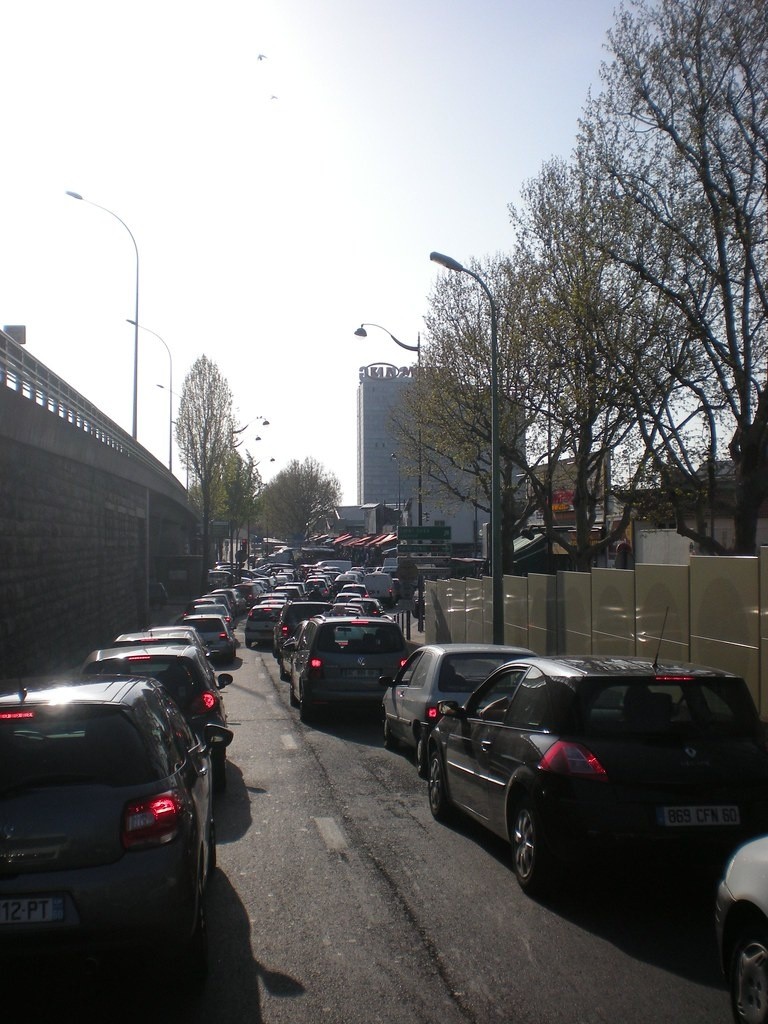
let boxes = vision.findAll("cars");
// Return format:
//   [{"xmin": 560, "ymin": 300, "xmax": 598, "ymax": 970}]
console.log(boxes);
[
  {"xmin": 113, "ymin": 632, "xmax": 216, "ymax": 677},
  {"xmin": 0, "ymin": 673, "xmax": 234, "ymax": 962},
  {"xmin": 74, "ymin": 645, "xmax": 234, "ymax": 797},
  {"xmin": 146, "ymin": 538, "xmax": 402, "ymax": 664},
  {"xmin": 710, "ymin": 837, "xmax": 768, "ymax": 1024},
  {"xmin": 377, "ymin": 642, "xmax": 540, "ymax": 779}
]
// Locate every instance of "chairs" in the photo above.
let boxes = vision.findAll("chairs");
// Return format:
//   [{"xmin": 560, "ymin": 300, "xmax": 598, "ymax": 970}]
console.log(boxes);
[{"xmin": 630, "ymin": 692, "xmax": 672, "ymax": 724}]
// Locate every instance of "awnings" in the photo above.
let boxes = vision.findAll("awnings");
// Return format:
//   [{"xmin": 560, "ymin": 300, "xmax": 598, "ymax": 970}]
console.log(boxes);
[
  {"xmin": 306, "ymin": 532, "xmax": 397, "ymax": 550},
  {"xmin": 512, "ymin": 531, "xmax": 546, "ymax": 554}
]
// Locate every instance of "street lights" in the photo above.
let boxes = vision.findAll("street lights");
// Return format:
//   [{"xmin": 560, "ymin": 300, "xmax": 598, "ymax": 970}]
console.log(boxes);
[
  {"xmin": 428, "ymin": 250, "xmax": 506, "ymax": 647},
  {"xmin": 64, "ymin": 187, "xmax": 141, "ymax": 442},
  {"xmin": 354, "ymin": 324, "xmax": 426, "ymax": 635},
  {"xmin": 155, "ymin": 383, "xmax": 190, "ymax": 497},
  {"xmin": 198, "ymin": 415, "xmax": 276, "ymax": 579},
  {"xmin": 127, "ymin": 318, "xmax": 172, "ymax": 472}
]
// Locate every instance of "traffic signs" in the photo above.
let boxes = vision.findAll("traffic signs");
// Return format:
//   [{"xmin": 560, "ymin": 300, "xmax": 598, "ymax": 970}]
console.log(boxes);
[{"xmin": 396, "ymin": 524, "xmax": 452, "ymax": 542}]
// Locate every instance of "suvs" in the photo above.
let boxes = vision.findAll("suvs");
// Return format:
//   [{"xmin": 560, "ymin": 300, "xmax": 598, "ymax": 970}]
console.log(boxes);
[
  {"xmin": 282, "ymin": 613, "xmax": 410, "ymax": 722},
  {"xmin": 421, "ymin": 652, "xmax": 768, "ymax": 902}
]
